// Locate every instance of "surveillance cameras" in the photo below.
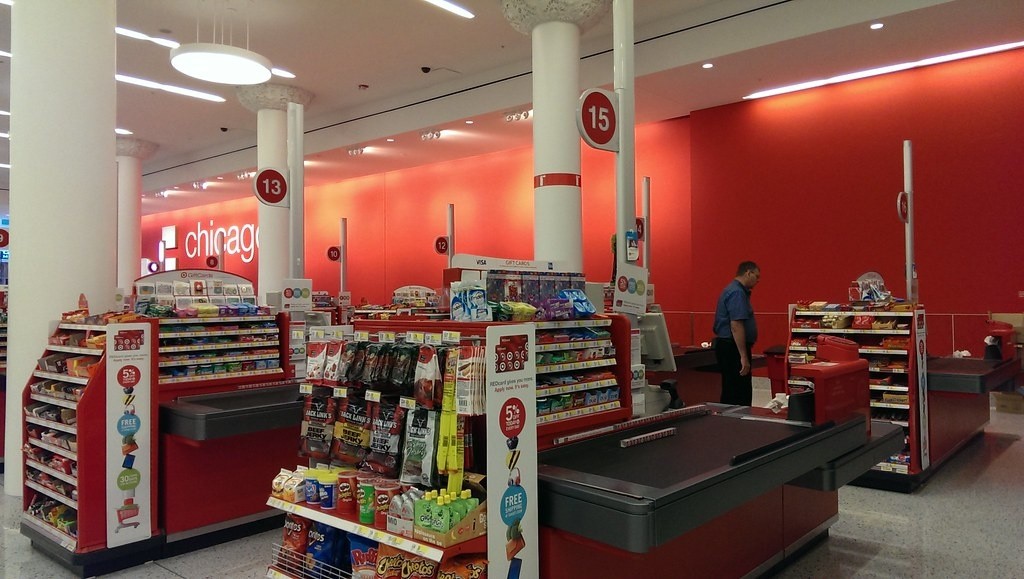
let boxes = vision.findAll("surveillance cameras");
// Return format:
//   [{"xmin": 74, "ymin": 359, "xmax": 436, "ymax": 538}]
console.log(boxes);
[
  {"xmin": 421, "ymin": 67, "xmax": 430, "ymax": 73},
  {"xmin": 221, "ymin": 128, "xmax": 227, "ymax": 132}
]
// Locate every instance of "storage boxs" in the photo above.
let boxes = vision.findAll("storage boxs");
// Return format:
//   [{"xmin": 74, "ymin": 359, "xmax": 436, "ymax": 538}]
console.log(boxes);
[
  {"xmin": 852, "ymin": 316, "xmax": 876, "ymax": 329},
  {"xmin": 791, "ymin": 337, "xmax": 809, "ymax": 346},
  {"xmin": 24, "ymin": 270, "xmax": 645, "ymax": 549},
  {"xmin": 988, "ymin": 310, "xmax": 1024, "ymax": 343},
  {"xmin": 788, "ymin": 355, "xmax": 806, "ymax": 363},
  {"xmin": 871, "ymin": 318, "xmax": 898, "ymax": 330},
  {"xmin": 889, "ymin": 304, "xmax": 917, "ymax": 312},
  {"xmin": 809, "ymin": 301, "xmax": 828, "ymax": 310},
  {"xmin": 889, "ymin": 455, "xmax": 910, "ymax": 463},
  {"xmin": 993, "ymin": 391, "xmax": 1024, "ymax": 414},
  {"xmin": 820, "ymin": 316, "xmax": 853, "ymax": 329},
  {"xmin": 883, "ymin": 391, "xmax": 909, "ymax": 404},
  {"xmin": 824, "ymin": 304, "xmax": 841, "ymax": 311},
  {"xmin": 882, "ymin": 336, "xmax": 911, "ymax": 350},
  {"xmin": 792, "ymin": 317, "xmax": 822, "ymax": 328},
  {"xmin": 840, "ymin": 303, "xmax": 852, "ymax": 311},
  {"xmin": 869, "ymin": 374, "xmax": 894, "ymax": 385},
  {"xmin": 850, "ymin": 301, "xmax": 871, "ymax": 312}
]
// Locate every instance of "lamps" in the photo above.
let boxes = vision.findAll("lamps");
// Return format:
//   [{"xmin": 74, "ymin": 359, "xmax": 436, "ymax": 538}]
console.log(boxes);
[{"xmin": 170, "ymin": 0, "xmax": 273, "ymax": 85}]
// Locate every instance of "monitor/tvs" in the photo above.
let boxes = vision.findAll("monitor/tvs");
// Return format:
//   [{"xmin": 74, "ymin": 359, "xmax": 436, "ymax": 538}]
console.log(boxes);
[
  {"xmin": 638, "ymin": 313, "xmax": 677, "ymax": 372},
  {"xmin": 305, "ymin": 311, "xmax": 330, "ymax": 327}
]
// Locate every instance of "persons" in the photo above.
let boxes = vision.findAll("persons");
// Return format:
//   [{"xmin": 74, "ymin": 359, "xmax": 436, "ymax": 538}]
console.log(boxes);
[{"xmin": 712, "ymin": 260, "xmax": 761, "ymax": 407}]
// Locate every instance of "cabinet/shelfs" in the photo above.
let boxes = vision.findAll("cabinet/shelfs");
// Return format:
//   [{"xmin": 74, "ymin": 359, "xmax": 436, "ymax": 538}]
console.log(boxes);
[
  {"xmin": 265, "ymin": 320, "xmax": 539, "ymax": 579},
  {"xmin": 0, "ymin": 284, "xmax": 8, "ymax": 475},
  {"xmin": 159, "ymin": 312, "xmax": 290, "ymax": 400},
  {"xmin": 538, "ymin": 315, "xmax": 633, "ymax": 439},
  {"xmin": 19, "ymin": 316, "xmax": 162, "ymax": 579},
  {"xmin": 784, "ymin": 303, "xmax": 933, "ymax": 496}
]
